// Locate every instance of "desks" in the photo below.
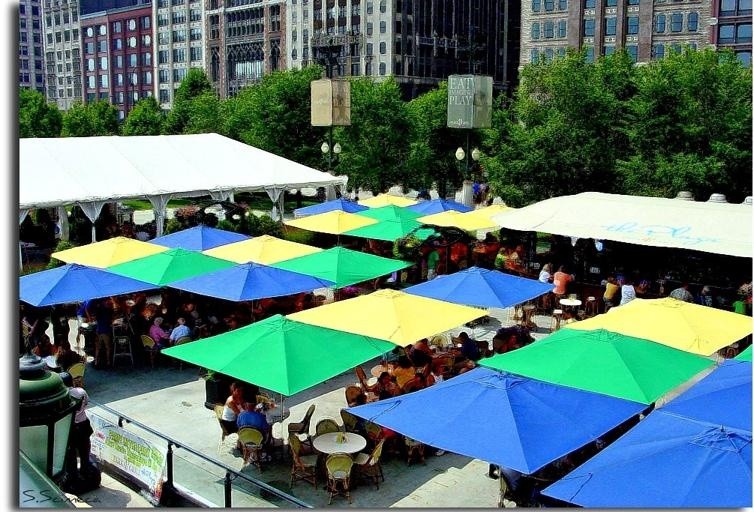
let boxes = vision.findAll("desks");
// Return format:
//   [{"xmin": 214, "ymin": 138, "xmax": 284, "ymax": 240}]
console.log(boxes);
[
  {"xmin": 19, "ymin": 334, "xmax": 78, "ymax": 497},
  {"xmin": 318, "ymin": 39, "xmax": 341, "ymax": 173},
  {"xmin": 455, "ymin": 28, "xmax": 486, "ymax": 207}
]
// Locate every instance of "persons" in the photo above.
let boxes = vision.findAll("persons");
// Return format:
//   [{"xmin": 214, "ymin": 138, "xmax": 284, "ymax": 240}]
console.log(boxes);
[
  {"xmin": 220, "ymin": 381, "xmax": 251, "ymax": 434},
  {"xmin": 58, "ymin": 371, "xmax": 95, "ymax": 474},
  {"xmin": 236, "ymin": 399, "xmax": 269, "ymax": 446},
  {"xmin": 345, "ymin": 233, "xmax": 536, "ymax": 395},
  {"xmin": 17, "ymin": 289, "xmax": 325, "ymax": 372},
  {"xmin": 552, "ymin": 235, "xmax": 752, "ymax": 317}
]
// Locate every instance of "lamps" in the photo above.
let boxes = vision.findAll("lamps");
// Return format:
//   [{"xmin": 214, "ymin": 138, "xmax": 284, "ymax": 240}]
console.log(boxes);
[
  {"xmin": 341, "ymin": 335, "xmax": 448, "ymax": 490},
  {"xmin": 286, "ymin": 404, "xmax": 316, "ymax": 457},
  {"xmin": 215, "ymin": 404, "xmax": 237, "ymax": 442},
  {"xmin": 325, "ymin": 452, "xmax": 354, "ymax": 505},
  {"xmin": 287, "ymin": 433, "xmax": 319, "ymax": 490},
  {"xmin": 316, "ymin": 419, "xmax": 340, "ymax": 436},
  {"xmin": 237, "ymin": 425, "xmax": 265, "ymax": 474},
  {"xmin": 141, "ymin": 335, "xmax": 158, "ymax": 371},
  {"xmin": 173, "ymin": 336, "xmax": 192, "ymax": 373},
  {"xmin": 239, "ymin": 404, "xmax": 290, "ymax": 465},
  {"xmin": 256, "ymin": 396, "xmax": 269, "ymax": 404}
]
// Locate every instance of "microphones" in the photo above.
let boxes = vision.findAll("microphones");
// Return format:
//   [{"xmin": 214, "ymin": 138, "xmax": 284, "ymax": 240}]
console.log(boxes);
[{"xmin": 585, "ymin": 296, "xmax": 599, "ymax": 317}]
